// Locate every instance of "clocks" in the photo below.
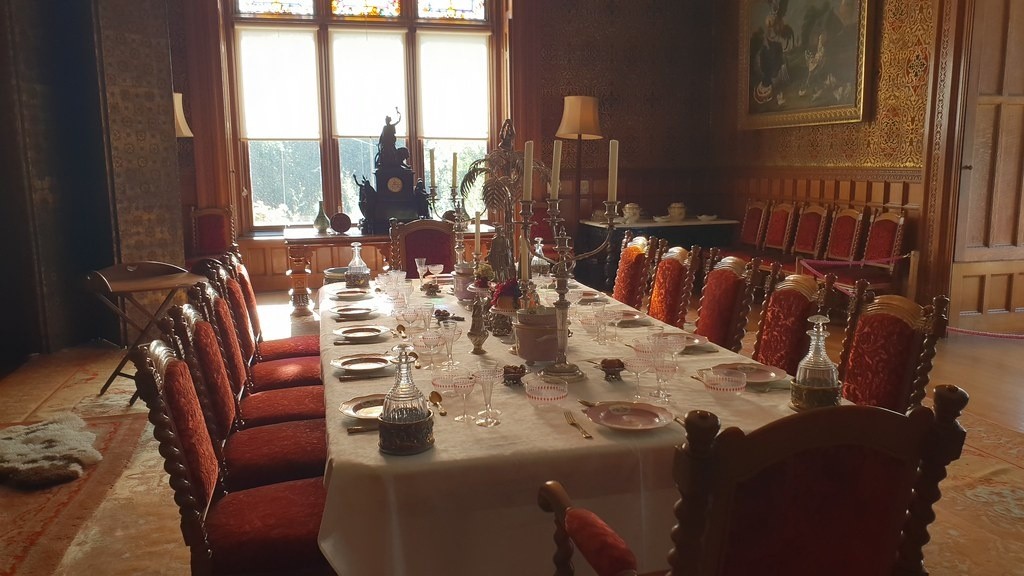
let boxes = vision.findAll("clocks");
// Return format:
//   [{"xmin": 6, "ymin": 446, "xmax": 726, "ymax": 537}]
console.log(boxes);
[{"xmin": 353, "ymin": 108, "xmax": 433, "ymax": 236}]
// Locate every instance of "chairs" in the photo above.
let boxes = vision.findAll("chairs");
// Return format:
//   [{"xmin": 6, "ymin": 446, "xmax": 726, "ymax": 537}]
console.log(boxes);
[{"xmin": 133, "ymin": 194, "xmax": 972, "ymax": 575}]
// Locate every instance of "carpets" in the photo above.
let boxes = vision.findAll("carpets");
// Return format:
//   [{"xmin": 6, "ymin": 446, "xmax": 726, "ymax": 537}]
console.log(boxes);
[{"xmin": 0, "ymin": 411, "xmax": 148, "ymax": 576}]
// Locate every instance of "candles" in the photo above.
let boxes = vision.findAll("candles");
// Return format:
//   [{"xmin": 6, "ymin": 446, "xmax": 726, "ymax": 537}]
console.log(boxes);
[
  {"xmin": 548, "ymin": 139, "xmax": 562, "ymax": 200},
  {"xmin": 605, "ymin": 139, "xmax": 621, "ymax": 200},
  {"xmin": 430, "ymin": 149, "xmax": 435, "ymax": 184},
  {"xmin": 520, "ymin": 227, "xmax": 530, "ymax": 281},
  {"xmin": 453, "ymin": 153, "xmax": 457, "ymax": 190},
  {"xmin": 521, "ymin": 141, "xmax": 534, "ymax": 202},
  {"xmin": 474, "ymin": 211, "xmax": 480, "ymax": 254}
]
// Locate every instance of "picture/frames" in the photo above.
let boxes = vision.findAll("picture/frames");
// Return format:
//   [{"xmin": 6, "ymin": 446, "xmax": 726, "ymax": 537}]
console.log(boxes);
[{"xmin": 736, "ymin": 0, "xmax": 875, "ymax": 131}]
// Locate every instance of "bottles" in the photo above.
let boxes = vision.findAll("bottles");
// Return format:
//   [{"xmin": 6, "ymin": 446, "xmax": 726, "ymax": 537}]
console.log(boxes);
[
  {"xmin": 380, "ymin": 343, "xmax": 431, "ymax": 455},
  {"xmin": 530, "ymin": 235, "xmax": 551, "ymax": 266},
  {"xmin": 347, "ymin": 241, "xmax": 368, "ymax": 283},
  {"xmin": 314, "ymin": 201, "xmax": 330, "ymax": 233},
  {"xmin": 796, "ymin": 314, "xmax": 839, "ymax": 407}
]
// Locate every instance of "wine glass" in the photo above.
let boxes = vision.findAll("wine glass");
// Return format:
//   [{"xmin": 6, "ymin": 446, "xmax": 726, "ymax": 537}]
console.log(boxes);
[
  {"xmin": 453, "ymin": 365, "xmax": 505, "ymax": 429},
  {"xmin": 428, "ymin": 261, "xmax": 444, "ymax": 290},
  {"xmin": 532, "ymin": 273, "xmax": 584, "ymax": 322},
  {"xmin": 374, "ymin": 268, "xmax": 414, "ymax": 303},
  {"xmin": 392, "ymin": 301, "xmax": 457, "ymax": 372},
  {"xmin": 414, "ymin": 256, "xmax": 427, "ymax": 286},
  {"xmin": 590, "ymin": 300, "xmax": 623, "ymax": 345},
  {"xmin": 621, "ymin": 326, "xmax": 686, "ymax": 400}
]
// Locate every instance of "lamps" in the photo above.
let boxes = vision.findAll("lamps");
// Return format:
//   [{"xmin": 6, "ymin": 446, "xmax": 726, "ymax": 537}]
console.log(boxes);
[
  {"xmin": 554, "ymin": 94, "xmax": 600, "ymax": 274},
  {"xmin": 173, "ymin": 90, "xmax": 193, "ymax": 138}
]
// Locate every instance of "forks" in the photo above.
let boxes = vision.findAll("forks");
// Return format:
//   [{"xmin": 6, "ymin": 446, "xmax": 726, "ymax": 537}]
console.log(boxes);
[{"xmin": 564, "ymin": 407, "xmax": 591, "ymax": 440}]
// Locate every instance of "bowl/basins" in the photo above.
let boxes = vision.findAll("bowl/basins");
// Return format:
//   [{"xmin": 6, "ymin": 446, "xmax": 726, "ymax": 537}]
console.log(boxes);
[
  {"xmin": 579, "ymin": 318, "xmax": 599, "ymax": 333},
  {"xmin": 700, "ymin": 368, "xmax": 746, "ymax": 401},
  {"xmin": 396, "ymin": 316, "xmax": 463, "ymax": 356},
  {"xmin": 525, "ymin": 376, "xmax": 569, "ymax": 408},
  {"xmin": 431, "ymin": 366, "xmax": 470, "ymax": 397}
]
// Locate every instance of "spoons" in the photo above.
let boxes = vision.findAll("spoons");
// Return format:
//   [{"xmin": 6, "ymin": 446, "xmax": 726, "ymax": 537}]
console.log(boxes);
[{"xmin": 428, "ymin": 389, "xmax": 447, "ymax": 416}]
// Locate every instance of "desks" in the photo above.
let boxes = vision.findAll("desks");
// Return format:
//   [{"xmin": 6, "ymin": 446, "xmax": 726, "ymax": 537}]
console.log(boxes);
[
  {"xmin": 283, "ymin": 219, "xmax": 494, "ymax": 317},
  {"xmin": 314, "ymin": 257, "xmax": 860, "ymax": 576},
  {"xmin": 90, "ymin": 258, "xmax": 209, "ymax": 404},
  {"xmin": 578, "ymin": 213, "xmax": 741, "ymax": 275}
]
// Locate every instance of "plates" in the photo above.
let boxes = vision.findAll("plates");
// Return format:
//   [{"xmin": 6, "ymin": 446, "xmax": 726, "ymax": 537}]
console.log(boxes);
[
  {"xmin": 425, "ymin": 274, "xmax": 455, "ymax": 281},
  {"xmin": 332, "ymin": 323, "xmax": 390, "ymax": 340},
  {"xmin": 581, "ymin": 397, "xmax": 677, "ymax": 431},
  {"xmin": 595, "ymin": 308, "xmax": 646, "ymax": 324},
  {"xmin": 647, "ymin": 332, "xmax": 709, "ymax": 348},
  {"xmin": 332, "ymin": 352, "xmax": 392, "ymax": 373},
  {"xmin": 333, "ymin": 289, "xmax": 370, "ymax": 300},
  {"xmin": 566, "ymin": 289, "xmax": 606, "ymax": 302},
  {"xmin": 333, "ymin": 306, "xmax": 373, "ymax": 319},
  {"xmin": 703, "ymin": 362, "xmax": 788, "ymax": 386},
  {"xmin": 338, "ymin": 393, "xmax": 393, "ymax": 420}
]
